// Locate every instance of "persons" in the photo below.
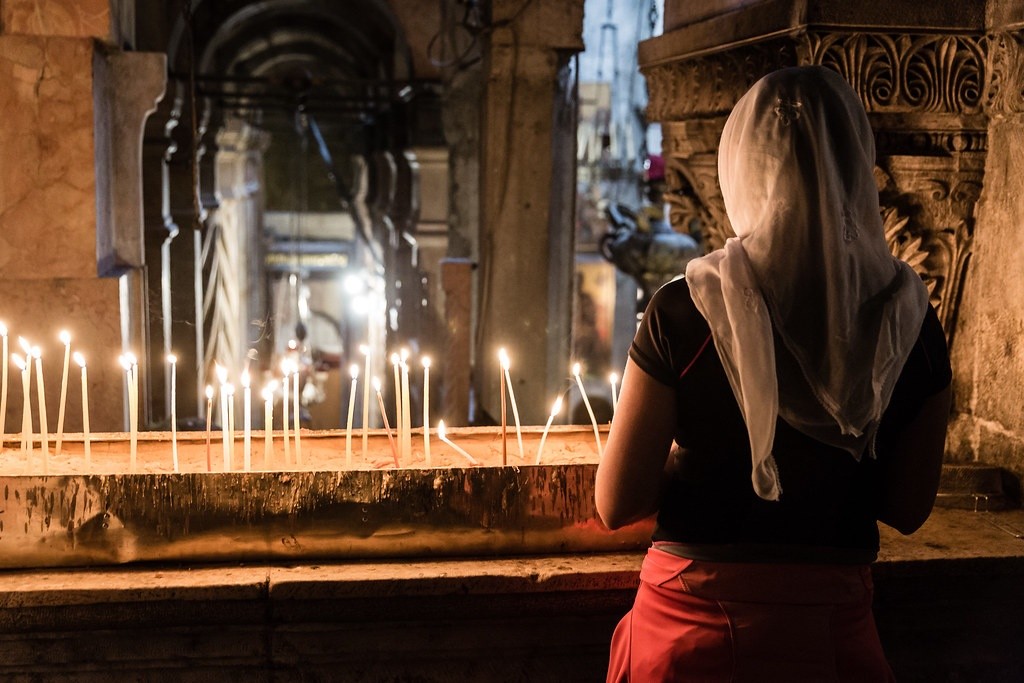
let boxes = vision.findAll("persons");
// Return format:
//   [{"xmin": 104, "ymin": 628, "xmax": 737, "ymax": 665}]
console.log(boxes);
[{"xmin": 594, "ymin": 64, "xmax": 954, "ymax": 683}]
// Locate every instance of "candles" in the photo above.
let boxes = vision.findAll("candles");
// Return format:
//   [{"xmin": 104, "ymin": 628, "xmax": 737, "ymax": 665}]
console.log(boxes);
[
  {"xmin": 11, "ymin": 353, "xmax": 34, "ymax": 454},
  {"xmin": 609, "ymin": 373, "xmax": 617, "ymax": 410},
  {"xmin": 31, "ymin": 344, "xmax": 48, "ymax": 454},
  {"xmin": 55, "ymin": 330, "xmax": 71, "ymax": 456},
  {"xmin": 0, "ymin": 322, "xmax": 8, "ymax": 453},
  {"xmin": 572, "ymin": 361, "xmax": 604, "ymax": 457},
  {"xmin": 73, "ymin": 350, "xmax": 91, "ymax": 458},
  {"xmin": 536, "ymin": 396, "xmax": 563, "ymax": 465},
  {"xmin": 204, "ymin": 346, "xmax": 479, "ymax": 472},
  {"xmin": 18, "ymin": 335, "xmax": 33, "ymax": 460},
  {"xmin": 168, "ymin": 354, "xmax": 180, "ymax": 472},
  {"xmin": 499, "ymin": 346, "xmax": 525, "ymax": 459},
  {"xmin": 119, "ymin": 352, "xmax": 139, "ymax": 463}
]
